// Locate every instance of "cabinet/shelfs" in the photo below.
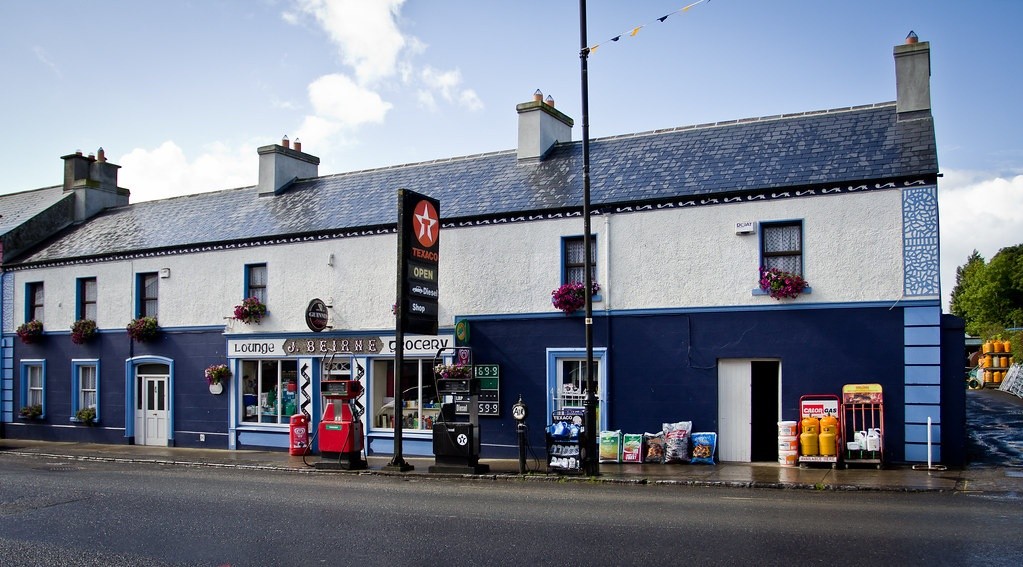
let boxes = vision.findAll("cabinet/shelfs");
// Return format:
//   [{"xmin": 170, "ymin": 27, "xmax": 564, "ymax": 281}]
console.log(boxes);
[{"xmin": 985, "ymin": 351, "xmax": 1014, "ymax": 388}]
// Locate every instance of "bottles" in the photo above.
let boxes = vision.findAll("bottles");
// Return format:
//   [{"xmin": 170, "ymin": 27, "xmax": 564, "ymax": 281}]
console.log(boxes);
[
  {"xmin": 867, "ymin": 434, "xmax": 879, "ymax": 451},
  {"xmin": 847, "ymin": 442, "xmax": 860, "ymax": 450},
  {"xmin": 407, "ymin": 412, "xmax": 413, "ymax": 428},
  {"xmin": 868, "ymin": 429, "xmax": 879, "ymax": 436},
  {"xmin": 414, "ymin": 411, "xmax": 418, "ymax": 428},
  {"xmin": 854, "ymin": 430, "xmax": 867, "ymax": 450}
]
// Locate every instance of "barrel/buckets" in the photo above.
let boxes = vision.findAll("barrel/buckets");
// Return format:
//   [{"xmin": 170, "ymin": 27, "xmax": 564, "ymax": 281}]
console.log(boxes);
[
  {"xmin": 777, "ymin": 421, "xmax": 796, "ymax": 435},
  {"xmin": 778, "ymin": 436, "xmax": 798, "ymax": 450},
  {"xmin": 778, "ymin": 451, "xmax": 798, "ymax": 467}
]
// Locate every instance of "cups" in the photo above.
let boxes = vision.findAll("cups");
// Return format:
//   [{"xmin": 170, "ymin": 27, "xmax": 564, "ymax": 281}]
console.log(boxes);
[
  {"xmin": 434, "ymin": 403, "xmax": 440, "ymax": 408},
  {"xmin": 381, "ymin": 414, "xmax": 388, "ymax": 428},
  {"xmin": 407, "ymin": 401, "xmax": 410, "ymax": 407},
  {"xmin": 410, "ymin": 401, "xmax": 415, "ymax": 407},
  {"xmin": 390, "ymin": 415, "xmax": 394, "ymax": 428},
  {"xmin": 376, "ymin": 414, "xmax": 382, "ymax": 428},
  {"xmin": 247, "ymin": 407, "xmax": 252, "ymax": 416},
  {"xmin": 402, "ymin": 401, "xmax": 406, "ymax": 407}
]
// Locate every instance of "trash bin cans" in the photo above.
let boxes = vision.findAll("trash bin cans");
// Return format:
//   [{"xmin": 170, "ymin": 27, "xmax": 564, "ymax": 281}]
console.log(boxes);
[{"xmin": 289, "ymin": 414, "xmax": 310, "ymax": 456}]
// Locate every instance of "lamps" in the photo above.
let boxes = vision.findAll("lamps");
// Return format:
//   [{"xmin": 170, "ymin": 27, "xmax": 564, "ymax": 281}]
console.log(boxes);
[{"xmin": 328, "ymin": 254, "xmax": 334, "ymax": 266}]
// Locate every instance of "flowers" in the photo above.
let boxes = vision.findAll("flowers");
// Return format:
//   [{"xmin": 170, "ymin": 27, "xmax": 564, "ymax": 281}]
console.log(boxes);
[
  {"xmin": 15, "ymin": 321, "xmax": 43, "ymax": 344},
  {"xmin": 757, "ymin": 267, "xmax": 807, "ymax": 302},
  {"xmin": 71, "ymin": 319, "xmax": 98, "ymax": 345},
  {"xmin": 434, "ymin": 363, "xmax": 473, "ymax": 380},
  {"xmin": 203, "ymin": 364, "xmax": 232, "ymax": 386},
  {"xmin": 233, "ymin": 298, "xmax": 267, "ymax": 323},
  {"xmin": 125, "ymin": 319, "xmax": 161, "ymax": 345},
  {"xmin": 552, "ymin": 279, "xmax": 586, "ymax": 315}
]
